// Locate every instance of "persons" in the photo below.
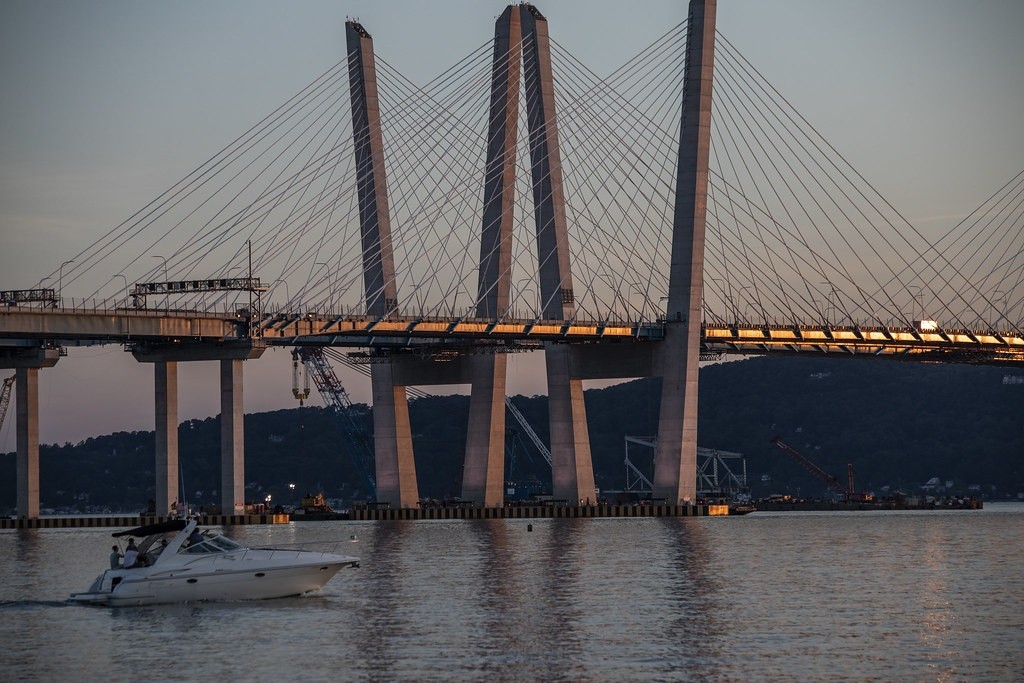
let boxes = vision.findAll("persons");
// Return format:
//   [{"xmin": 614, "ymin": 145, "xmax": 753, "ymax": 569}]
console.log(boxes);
[
  {"xmin": 187, "ymin": 527, "xmax": 205, "ymax": 553},
  {"xmin": 199, "ymin": 504, "xmax": 204, "ymax": 515},
  {"xmin": 110, "ymin": 538, "xmax": 169, "ymax": 569}
]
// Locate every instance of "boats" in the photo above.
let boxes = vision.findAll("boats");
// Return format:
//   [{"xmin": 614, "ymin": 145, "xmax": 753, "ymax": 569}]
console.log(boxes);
[
  {"xmin": 65, "ymin": 461, "xmax": 360, "ymax": 603},
  {"xmin": 698, "ymin": 497, "xmax": 757, "ymax": 516}
]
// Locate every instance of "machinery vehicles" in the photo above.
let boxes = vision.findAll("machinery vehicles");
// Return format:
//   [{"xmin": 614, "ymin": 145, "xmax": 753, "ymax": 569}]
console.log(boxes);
[
  {"xmin": 300, "ymin": 492, "xmax": 336, "ymax": 512},
  {"xmin": 505, "ymin": 393, "xmax": 601, "ymax": 500},
  {"xmin": 0, "ymin": 372, "xmax": 18, "ymax": 432},
  {"xmin": 290, "ymin": 341, "xmax": 377, "ymax": 502},
  {"xmin": 769, "ymin": 436, "xmax": 873, "ymax": 501}
]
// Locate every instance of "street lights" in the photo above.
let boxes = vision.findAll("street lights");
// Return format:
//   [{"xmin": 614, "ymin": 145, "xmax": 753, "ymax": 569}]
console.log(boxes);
[
  {"xmin": 811, "ymin": 283, "xmax": 841, "ymax": 327},
  {"xmin": 151, "ymin": 256, "xmax": 169, "ymax": 309},
  {"xmin": 59, "ymin": 261, "xmax": 75, "ymax": 309},
  {"xmin": 890, "ymin": 285, "xmax": 926, "ymax": 327},
  {"xmin": 246, "ymin": 240, "xmax": 255, "ymax": 339},
  {"xmin": 315, "ymin": 262, "xmax": 332, "ymax": 309},
  {"xmin": 338, "ymin": 288, "xmax": 348, "ymax": 315},
  {"xmin": 273, "ymin": 279, "xmax": 289, "ymax": 304},
  {"xmin": 113, "ymin": 274, "xmax": 129, "ymax": 308},
  {"xmin": 226, "ymin": 266, "xmax": 244, "ymax": 311},
  {"xmin": 39, "ymin": 278, "xmax": 50, "ymax": 297},
  {"xmin": 966, "ymin": 289, "xmax": 1012, "ymax": 334},
  {"xmin": 387, "ymin": 268, "xmax": 755, "ymax": 326}
]
